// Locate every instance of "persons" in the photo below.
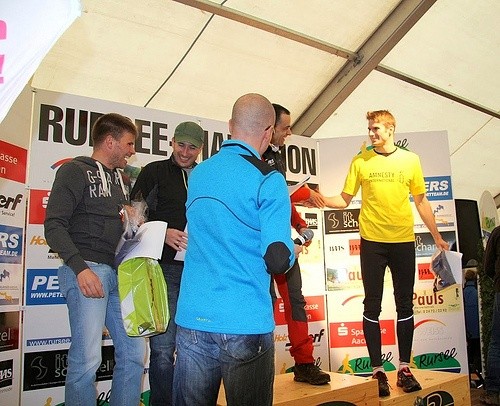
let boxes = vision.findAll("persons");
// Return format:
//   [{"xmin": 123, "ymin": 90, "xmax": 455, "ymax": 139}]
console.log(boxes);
[
  {"xmin": 265, "ymin": 104, "xmax": 325, "ymax": 386},
  {"xmin": 463, "ymin": 270, "xmax": 484, "ymax": 388},
  {"xmin": 43, "ymin": 113, "xmax": 147, "ymax": 406},
  {"xmin": 308, "ymin": 109, "xmax": 452, "ymax": 396},
  {"xmin": 170, "ymin": 93, "xmax": 304, "ymax": 406},
  {"xmin": 130, "ymin": 122, "xmax": 206, "ymax": 406},
  {"xmin": 479, "ymin": 226, "xmax": 500, "ymax": 406}
]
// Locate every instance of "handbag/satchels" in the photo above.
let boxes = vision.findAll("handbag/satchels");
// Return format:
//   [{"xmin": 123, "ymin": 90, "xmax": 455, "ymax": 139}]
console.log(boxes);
[{"xmin": 118, "ymin": 258, "xmax": 169, "ymax": 338}]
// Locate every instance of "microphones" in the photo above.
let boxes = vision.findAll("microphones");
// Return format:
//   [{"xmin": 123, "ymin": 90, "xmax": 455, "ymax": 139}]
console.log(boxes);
[{"xmin": 294, "ymin": 229, "xmax": 314, "ymax": 245}]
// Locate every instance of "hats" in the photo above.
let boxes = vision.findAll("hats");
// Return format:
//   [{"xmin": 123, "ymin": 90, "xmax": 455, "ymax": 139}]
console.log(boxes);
[{"xmin": 175, "ymin": 122, "xmax": 205, "ymax": 148}]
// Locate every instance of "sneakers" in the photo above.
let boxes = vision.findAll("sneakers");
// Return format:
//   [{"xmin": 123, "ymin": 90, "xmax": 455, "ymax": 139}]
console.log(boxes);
[
  {"xmin": 294, "ymin": 357, "xmax": 330, "ymax": 385},
  {"xmin": 397, "ymin": 367, "xmax": 422, "ymax": 393},
  {"xmin": 372, "ymin": 371, "xmax": 390, "ymax": 397}
]
